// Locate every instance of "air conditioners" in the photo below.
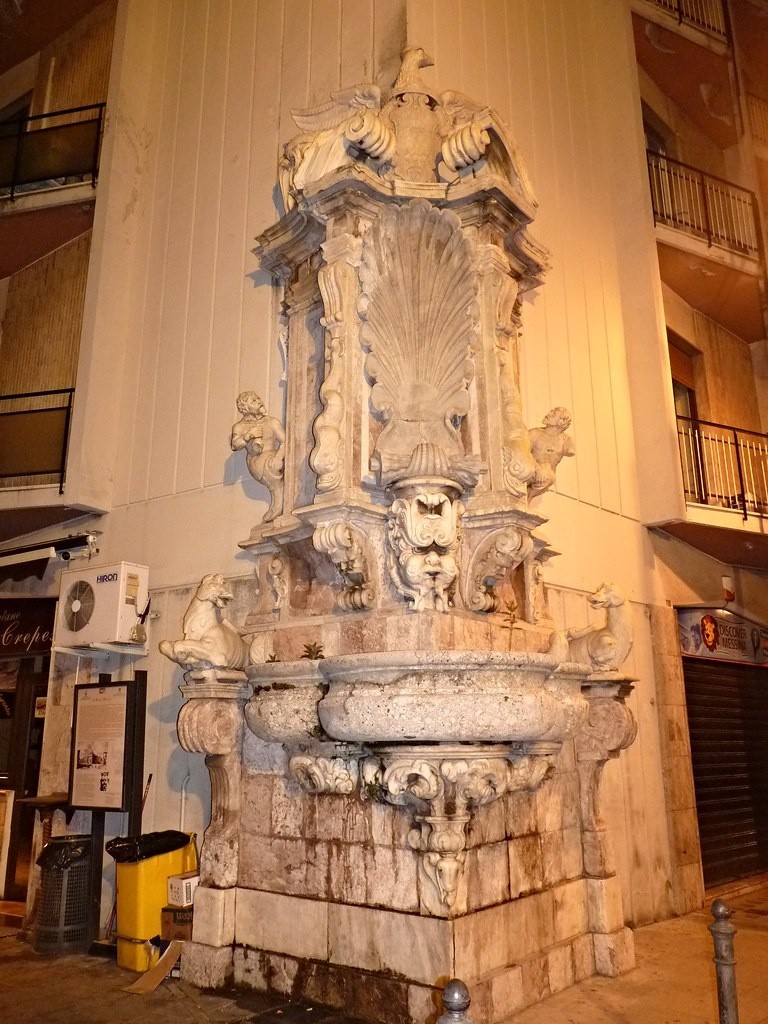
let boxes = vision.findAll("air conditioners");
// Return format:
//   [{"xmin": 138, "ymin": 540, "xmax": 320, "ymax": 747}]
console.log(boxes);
[{"xmin": 53, "ymin": 560, "xmax": 150, "ymax": 649}]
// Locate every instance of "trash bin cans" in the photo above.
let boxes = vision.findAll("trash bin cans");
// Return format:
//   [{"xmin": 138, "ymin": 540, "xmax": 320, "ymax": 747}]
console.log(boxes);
[
  {"xmin": 109, "ymin": 829, "xmax": 199, "ymax": 973},
  {"xmin": 33, "ymin": 833, "xmax": 92, "ymax": 957}
]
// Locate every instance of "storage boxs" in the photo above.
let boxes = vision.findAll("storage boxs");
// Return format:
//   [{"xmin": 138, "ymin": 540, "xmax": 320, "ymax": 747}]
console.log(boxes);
[
  {"xmin": 161, "ymin": 906, "xmax": 192, "ymax": 942},
  {"xmin": 167, "ymin": 870, "xmax": 200, "ymax": 907}
]
